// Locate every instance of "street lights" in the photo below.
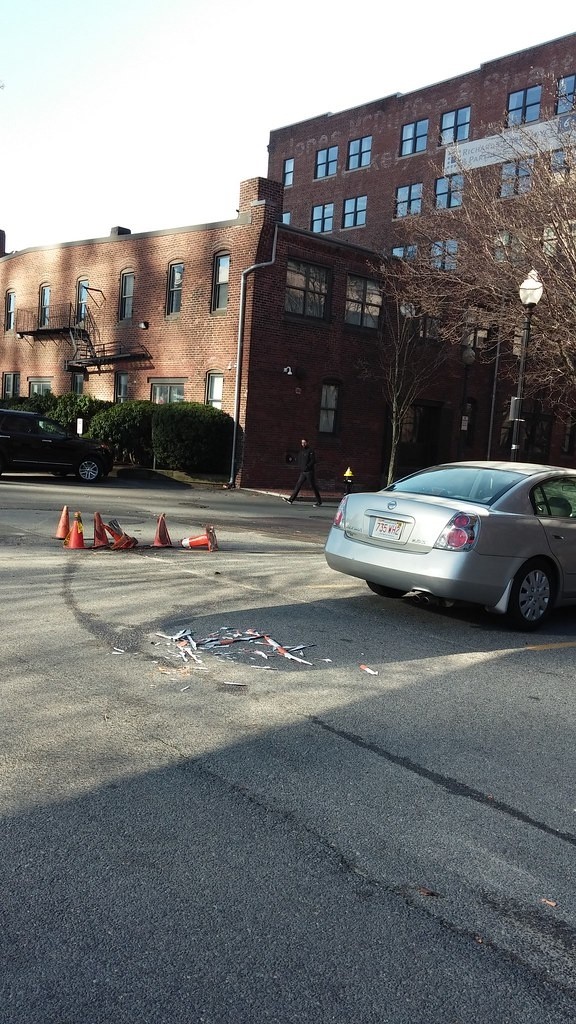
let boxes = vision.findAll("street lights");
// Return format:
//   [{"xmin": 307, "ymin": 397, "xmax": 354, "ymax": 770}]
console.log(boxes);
[{"xmin": 511, "ymin": 268, "xmax": 543, "ymax": 462}]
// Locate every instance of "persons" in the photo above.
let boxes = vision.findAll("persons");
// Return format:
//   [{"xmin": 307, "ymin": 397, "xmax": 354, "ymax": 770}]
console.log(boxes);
[{"xmin": 282, "ymin": 439, "xmax": 323, "ymax": 507}]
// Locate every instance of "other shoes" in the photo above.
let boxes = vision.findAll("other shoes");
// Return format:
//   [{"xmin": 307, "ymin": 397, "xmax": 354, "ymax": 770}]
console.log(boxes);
[
  {"xmin": 282, "ymin": 497, "xmax": 293, "ymax": 504},
  {"xmin": 313, "ymin": 501, "xmax": 322, "ymax": 507}
]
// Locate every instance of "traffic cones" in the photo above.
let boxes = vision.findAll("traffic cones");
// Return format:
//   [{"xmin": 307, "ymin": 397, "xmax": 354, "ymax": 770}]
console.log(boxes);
[
  {"xmin": 105, "ymin": 526, "xmax": 138, "ymax": 550},
  {"xmin": 63, "ymin": 511, "xmax": 90, "ymax": 549},
  {"xmin": 91, "ymin": 511, "xmax": 110, "ymax": 548},
  {"xmin": 52, "ymin": 505, "xmax": 69, "ymax": 539},
  {"xmin": 181, "ymin": 524, "xmax": 219, "ymax": 552},
  {"xmin": 150, "ymin": 512, "xmax": 174, "ymax": 548}
]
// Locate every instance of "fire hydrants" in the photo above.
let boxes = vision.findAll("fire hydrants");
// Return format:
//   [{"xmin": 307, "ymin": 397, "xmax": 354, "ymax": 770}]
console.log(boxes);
[{"xmin": 343, "ymin": 467, "xmax": 354, "ymax": 497}]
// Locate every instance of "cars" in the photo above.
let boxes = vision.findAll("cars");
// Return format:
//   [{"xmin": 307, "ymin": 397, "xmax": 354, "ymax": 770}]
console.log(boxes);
[
  {"xmin": 0, "ymin": 409, "xmax": 113, "ymax": 482},
  {"xmin": 325, "ymin": 460, "xmax": 576, "ymax": 629}
]
